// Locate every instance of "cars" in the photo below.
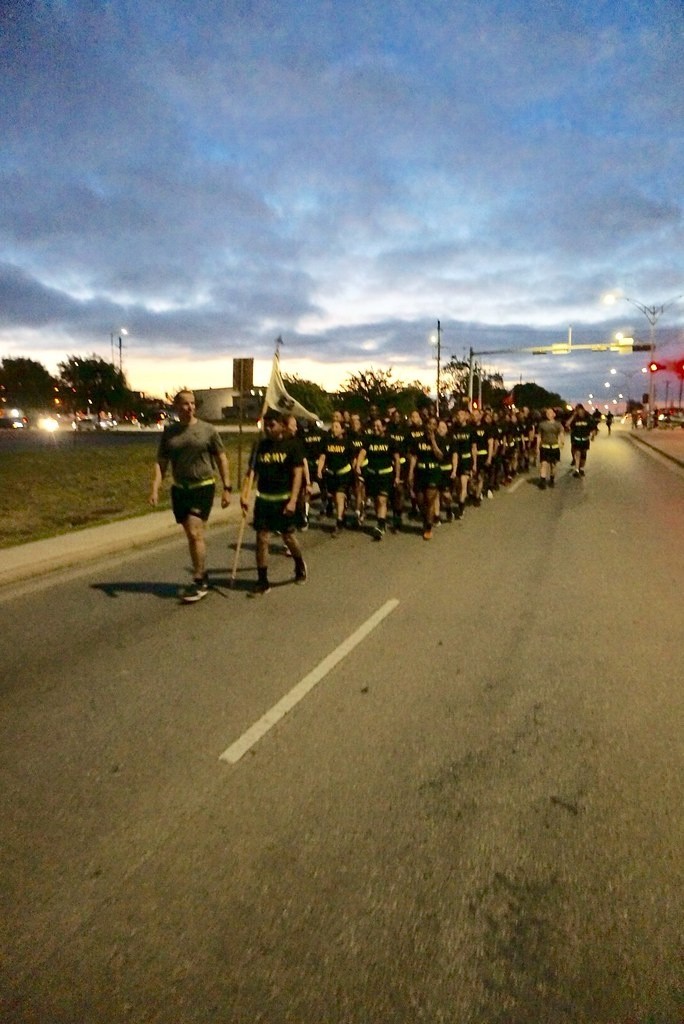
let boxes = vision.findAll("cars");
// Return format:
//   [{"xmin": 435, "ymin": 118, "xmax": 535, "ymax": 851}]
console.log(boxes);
[{"xmin": 0, "ymin": 406, "xmax": 116, "ymax": 431}]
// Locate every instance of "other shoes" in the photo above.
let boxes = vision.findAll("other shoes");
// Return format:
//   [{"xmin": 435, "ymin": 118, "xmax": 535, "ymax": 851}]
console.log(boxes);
[
  {"xmin": 538, "ymin": 481, "xmax": 547, "ymax": 489},
  {"xmin": 458, "ymin": 507, "xmax": 465, "ymax": 519},
  {"xmin": 300, "ymin": 518, "xmax": 308, "ymax": 531},
  {"xmin": 373, "ymin": 527, "xmax": 383, "ymax": 540},
  {"xmin": 393, "ymin": 519, "xmax": 403, "ymax": 534},
  {"xmin": 423, "ymin": 525, "xmax": 433, "ymax": 539},
  {"xmin": 434, "ymin": 517, "xmax": 442, "ymax": 528},
  {"xmin": 295, "ymin": 562, "xmax": 307, "ymax": 583},
  {"xmin": 246, "ymin": 581, "xmax": 270, "ymax": 598},
  {"xmin": 447, "ymin": 507, "xmax": 455, "ymax": 523},
  {"xmin": 333, "ymin": 527, "xmax": 345, "ymax": 537},
  {"xmin": 473, "ymin": 495, "xmax": 483, "ymax": 509},
  {"xmin": 548, "ymin": 480, "xmax": 555, "ymax": 487},
  {"xmin": 182, "ymin": 578, "xmax": 211, "ymax": 601},
  {"xmin": 573, "ymin": 471, "xmax": 580, "ymax": 478},
  {"xmin": 579, "ymin": 470, "xmax": 585, "ymax": 476}
]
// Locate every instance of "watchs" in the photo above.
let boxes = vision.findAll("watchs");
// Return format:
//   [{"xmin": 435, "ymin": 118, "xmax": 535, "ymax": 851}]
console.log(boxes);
[{"xmin": 223, "ymin": 484, "xmax": 235, "ymax": 492}]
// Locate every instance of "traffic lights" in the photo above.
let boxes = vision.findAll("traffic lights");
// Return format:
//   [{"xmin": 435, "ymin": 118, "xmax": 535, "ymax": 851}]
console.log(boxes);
[{"xmin": 648, "ymin": 363, "xmax": 659, "ymax": 373}]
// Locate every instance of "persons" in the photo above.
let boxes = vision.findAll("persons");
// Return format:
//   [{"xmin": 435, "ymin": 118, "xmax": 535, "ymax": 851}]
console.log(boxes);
[
  {"xmin": 240, "ymin": 404, "xmax": 613, "ymax": 597},
  {"xmin": 631, "ymin": 408, "xmax": 673, "ymax": 429},
  {"xmin": 147, "ymin": 391, "xmax": 232, "ymax": 602}
]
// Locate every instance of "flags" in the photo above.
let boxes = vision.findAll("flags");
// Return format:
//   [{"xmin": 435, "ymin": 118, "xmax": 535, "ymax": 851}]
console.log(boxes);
[{"xmin": 265, "ymin": 349, "xmax": 319, "ymax": 422}]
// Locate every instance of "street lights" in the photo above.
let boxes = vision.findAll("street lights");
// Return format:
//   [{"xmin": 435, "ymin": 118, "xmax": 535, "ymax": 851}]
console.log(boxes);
[
  {"xmin": 118, "ymin": 329, "xmax": 129, "ymax": 372},
  {"xmin": 603, "ymin": 291, "xmax": 684, "ymax": 431}
]
